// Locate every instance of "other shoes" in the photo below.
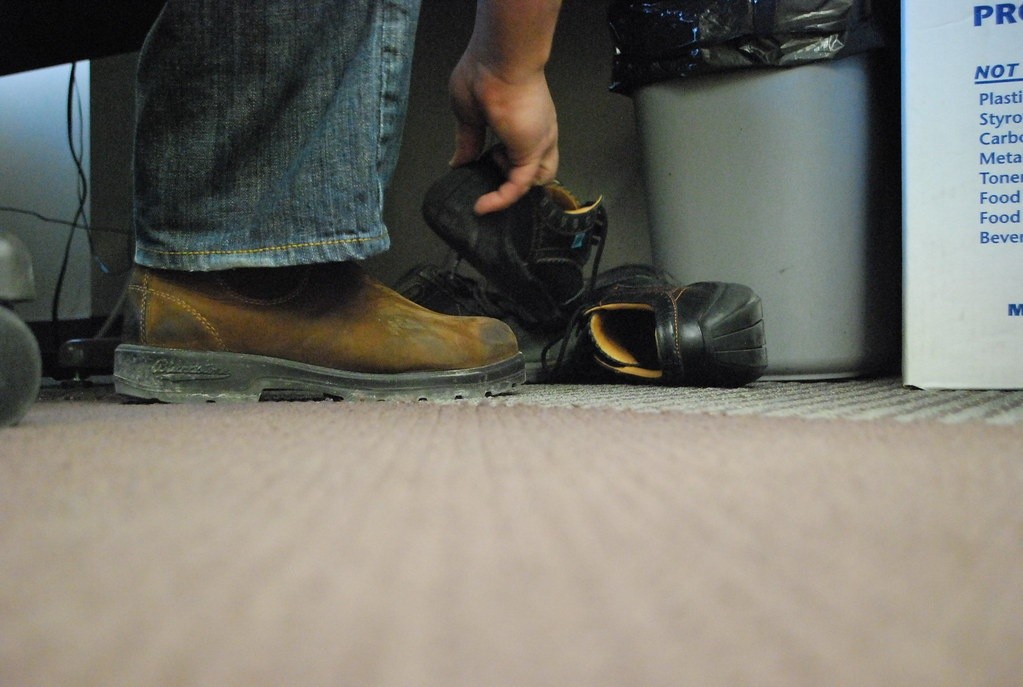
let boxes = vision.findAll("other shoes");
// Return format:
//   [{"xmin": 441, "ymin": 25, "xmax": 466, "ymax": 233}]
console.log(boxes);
[{"xmin": 116, "ymin": 253, "xmax": 527, "ymax": 403}]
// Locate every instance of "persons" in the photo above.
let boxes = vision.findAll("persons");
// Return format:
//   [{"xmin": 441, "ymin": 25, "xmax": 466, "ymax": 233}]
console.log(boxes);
[{"xmin": 0, "ymin": 0, "xmax": 563, "ymax": 403}]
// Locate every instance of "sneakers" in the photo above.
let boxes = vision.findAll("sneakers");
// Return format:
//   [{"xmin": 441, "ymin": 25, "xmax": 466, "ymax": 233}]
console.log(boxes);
[
  {"xmin": 395, "ymin": 262, "xmax": 564, "ymax": 383},
  {"xmin": 423, "ymin": 144, "xmax": 608, "ymax": 329},
  {"xmin": 575, "ymin": 264, "xmax": 768, "ymax": 386}
]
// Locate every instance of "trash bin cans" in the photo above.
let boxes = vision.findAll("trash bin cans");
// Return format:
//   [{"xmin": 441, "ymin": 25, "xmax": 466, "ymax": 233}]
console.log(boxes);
[{"xmin": 628, "ymin": 0, "xmax": 873, "ymax": 382}]
